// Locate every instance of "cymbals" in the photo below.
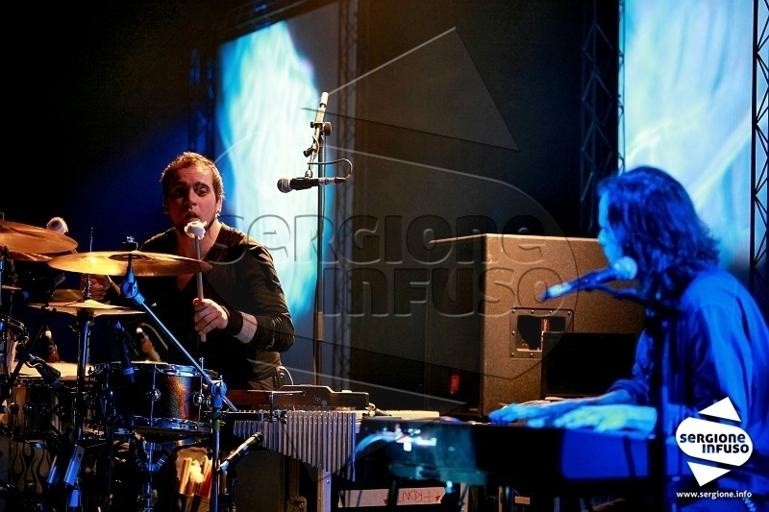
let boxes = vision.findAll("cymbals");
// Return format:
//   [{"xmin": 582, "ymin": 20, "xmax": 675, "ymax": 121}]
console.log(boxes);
[
  {"xmin": 48, "ymin": 250, "xmax": 213, "ymax": 276},
  {"xmin": 0, "ymin": 220, "xmax": 78, "ymax": 262},
  {"xmin": 29, "ymin": 300, "xmax": 147, "ymax": 317}
]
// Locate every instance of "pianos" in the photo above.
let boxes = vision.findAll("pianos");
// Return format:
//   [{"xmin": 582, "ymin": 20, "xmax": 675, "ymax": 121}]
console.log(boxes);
[{"xmin": 233, "ymin": 407, "xmax": 716, "ymax": 484}]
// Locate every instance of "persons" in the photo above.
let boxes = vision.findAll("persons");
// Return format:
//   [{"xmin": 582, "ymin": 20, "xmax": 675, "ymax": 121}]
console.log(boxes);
[
  {"xmin": 487, "ymin": 166, "xmax": 769, "ymax": 511},
  {"xmin": 80, "ymin": 153, "xmax": 296, "ymax": 512}
]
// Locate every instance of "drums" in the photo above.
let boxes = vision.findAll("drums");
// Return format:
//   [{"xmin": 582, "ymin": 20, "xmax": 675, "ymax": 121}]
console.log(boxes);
[
  {"xmin": 43, "ymin": 440, "xmax": 174, "ymax": 512},
  {"xmin": 109, "ymin": 361, "xmax": 213, "ymax": 434},
  {"xmin": 2, "ymin": 362, "xmax": 99, "ymax": 441}
]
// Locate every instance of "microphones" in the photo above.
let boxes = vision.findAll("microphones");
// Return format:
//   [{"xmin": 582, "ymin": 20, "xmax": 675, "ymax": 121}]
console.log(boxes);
[
  {"xmin": 220, "ymin": 432, "xmax": 263, "ymax": 471},
  {"xmin": 542, "ymin": 256, "xmax": 638, "ymax": 300},
  {"xmin": 278, "ymin": 176, "xmax": 336, "ymax": 194}
]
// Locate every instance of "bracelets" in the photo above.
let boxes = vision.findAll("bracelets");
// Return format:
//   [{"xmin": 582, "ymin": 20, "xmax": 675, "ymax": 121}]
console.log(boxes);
[{"xmin": 234, "ymin": 309, "xmax": 259, "ymax": 344}]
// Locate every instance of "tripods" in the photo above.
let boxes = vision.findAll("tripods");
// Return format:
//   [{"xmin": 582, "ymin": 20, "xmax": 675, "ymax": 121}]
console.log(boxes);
[{"xmin": 0, "ymin": 278, "xmax": 239, "ymax": 511}]
